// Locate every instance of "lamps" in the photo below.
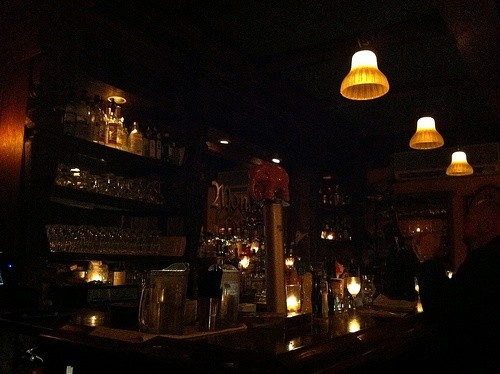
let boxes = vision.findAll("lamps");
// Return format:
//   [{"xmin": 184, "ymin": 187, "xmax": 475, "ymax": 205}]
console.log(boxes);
[
  {"xmin": 446, "ymin": 146, "xmax": 473, "ymax": 176},
  {"xmin": 340, "ymin": 50, "xmax": 390, "ymax": 101},
  {"xmin": 409, "ymin": 117, "xmax": 445, "ymax": 150}
]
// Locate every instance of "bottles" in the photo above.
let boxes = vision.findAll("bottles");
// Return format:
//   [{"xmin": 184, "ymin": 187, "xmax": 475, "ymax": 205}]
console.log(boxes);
[
  {"xmin": 200, "ymin": 227, "xmax": 265, "ymax": 257},
  {"xmin": 65, "ymin": 90, "xmax": 184, "ymax": 163},
  {"xmin": 319, "ymin": 184, "xmax": 348, "ymax": 204}
]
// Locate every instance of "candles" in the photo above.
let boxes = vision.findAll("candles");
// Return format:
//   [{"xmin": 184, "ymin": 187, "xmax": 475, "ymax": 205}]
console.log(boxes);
[
  {"xmin": 287, "ymin": 295, "xmax": 298, "ymax": 309},
  {"xmin": 348, "ymin": 280, "xmax": 360, "ymax": 294}
]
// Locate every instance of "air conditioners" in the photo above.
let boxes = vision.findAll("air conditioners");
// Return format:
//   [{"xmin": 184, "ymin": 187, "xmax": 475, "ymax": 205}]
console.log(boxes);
[{"xmin": 393, "ymin": 142, "xmax": 500, "ymax": 182}]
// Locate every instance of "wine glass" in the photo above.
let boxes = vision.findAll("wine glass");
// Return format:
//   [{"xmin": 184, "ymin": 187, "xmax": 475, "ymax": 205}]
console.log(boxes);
[
  {"xmin": 361, "ymin": 275, "xmax": 375, "ymax": 307},
  {"xmin": 347, "ymin": 277, "xmax": 361, "ymax": 311}
]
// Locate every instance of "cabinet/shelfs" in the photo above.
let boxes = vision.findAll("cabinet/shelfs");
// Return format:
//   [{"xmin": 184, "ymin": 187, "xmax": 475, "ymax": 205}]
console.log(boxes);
[{"xmin": 24, "ymin": 128, "xmax": 203, "ymax": 263}]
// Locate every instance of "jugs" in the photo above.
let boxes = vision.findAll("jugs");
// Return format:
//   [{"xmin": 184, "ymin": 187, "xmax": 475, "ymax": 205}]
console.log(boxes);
[{"xmin": 137, "ymin": 262, "xmax": 191, "ymax": 334}]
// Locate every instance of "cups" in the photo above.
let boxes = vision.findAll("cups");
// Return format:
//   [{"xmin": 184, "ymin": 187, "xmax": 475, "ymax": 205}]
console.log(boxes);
[
  {"xmin": 45, "ymin": 224, "xmax": 160, "ymax": 255},
  {"xmin": 58, "ymin": 165, "xmax": 160, "ymax": 202}
]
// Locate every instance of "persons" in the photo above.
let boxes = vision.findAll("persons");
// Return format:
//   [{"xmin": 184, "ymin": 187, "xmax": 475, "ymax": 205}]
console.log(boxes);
[
  {"xmin": 416, "ymin": 200, "xmax": 500, "ymax": 374},
  {"xmin": 382, "ymin": 222, "xmax": 419, "ymax": 300},
  {"xmin": 321, "ymin": 241, "xmax": 359, "ymax": 279}
]
